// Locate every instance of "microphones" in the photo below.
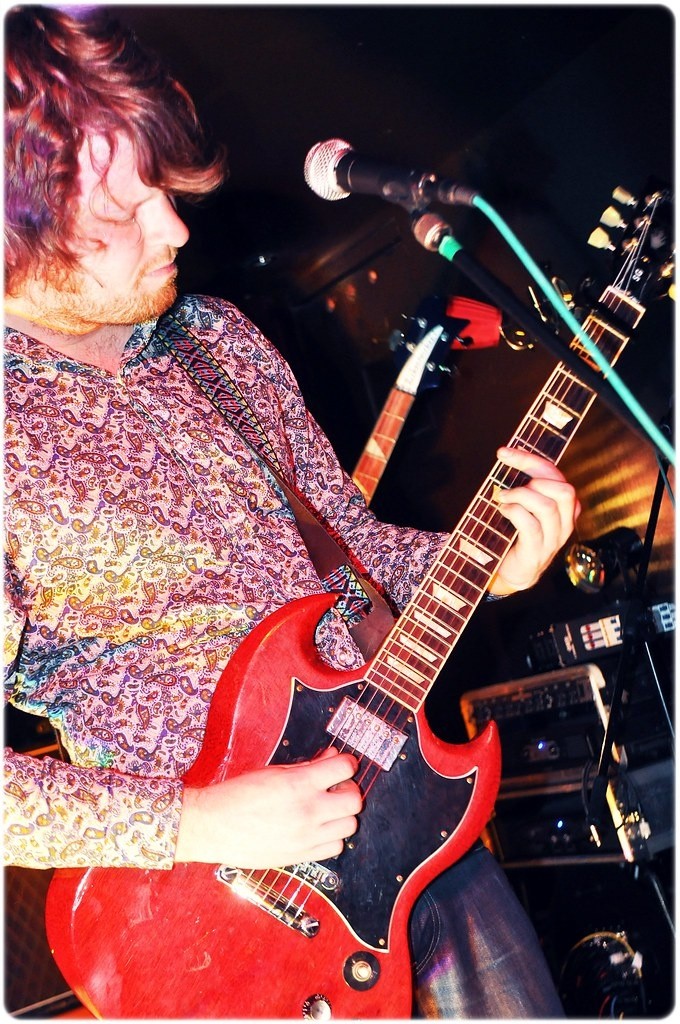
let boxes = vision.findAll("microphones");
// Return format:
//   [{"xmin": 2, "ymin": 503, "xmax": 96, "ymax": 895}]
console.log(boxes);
[{"xmin": 303, "ymin": 138, "xmax": 480, "ymax": 208}]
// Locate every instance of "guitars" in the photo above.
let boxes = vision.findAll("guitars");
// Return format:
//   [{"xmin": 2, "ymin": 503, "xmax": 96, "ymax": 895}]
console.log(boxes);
[
  {"xmin": 38, "ymin": 187, "xmax": 675, "ymax": 1019},
  {"xmin": 349, "ymin": 312, "xmax": 474, "ymax": 510}
]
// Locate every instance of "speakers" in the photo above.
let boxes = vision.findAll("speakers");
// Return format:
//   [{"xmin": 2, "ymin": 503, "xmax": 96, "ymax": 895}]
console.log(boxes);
[{"xmin": 503, "ymin": 846, "xmax": 676, "ymax": 1020}]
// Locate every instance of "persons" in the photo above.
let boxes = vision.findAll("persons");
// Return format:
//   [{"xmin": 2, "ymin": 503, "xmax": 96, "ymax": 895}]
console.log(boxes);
[{"xmin": 4, "ymin": 7, "xmax": 584, "ymax": 1020}]
